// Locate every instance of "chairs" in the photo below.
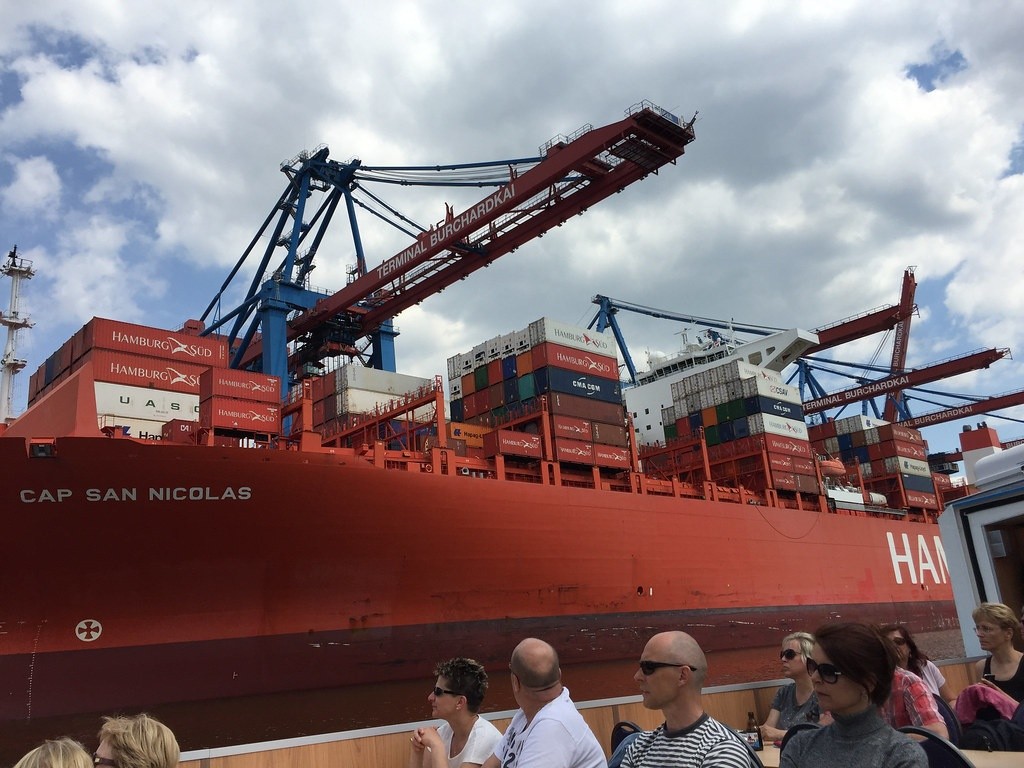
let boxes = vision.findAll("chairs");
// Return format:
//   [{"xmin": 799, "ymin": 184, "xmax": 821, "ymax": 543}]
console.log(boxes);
[
  {"xmin": 956, "ymin": 683, "xmax": 1016, "ymax": 726},
  {"xmin": 896, "ymin": 726, "xmax": 976, "ymax": 768},
  {"xmin": 932, "ymin": 692, "xmax": 964, "ymax": 748},
  {"xmin": 610, "ymin": 720, "xmax": 643, "ymax": 755}
]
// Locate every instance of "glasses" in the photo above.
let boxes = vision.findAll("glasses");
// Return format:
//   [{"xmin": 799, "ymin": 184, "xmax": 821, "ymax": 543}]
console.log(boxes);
[
  {"xmin": 806, "ymin": 657, "xmax": 846, "ymax": 684},
  {"xmin": 780, "ymin": 649, "xmax": 801, "ymax": 660},
  {"xmin": 432, "ymin": 687, "xmax": 471, "ymax": 704},
  {"xmin": 640, "ymin": 661, "xmax": 697, "ymax": 675},
  {"xmin": 92, "ymin": 752, "xmax": 116, "ymax": 768},
  {"xmin": 893, "ymin": 637, "xmax": 906, "ymax": 645},
  {"xmin": 973, "ymin": 626, "xmax": 1015, "ymax": 634}
]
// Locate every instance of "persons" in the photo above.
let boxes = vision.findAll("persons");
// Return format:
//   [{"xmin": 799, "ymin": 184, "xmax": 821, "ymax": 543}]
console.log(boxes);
[
  {"xmin": 481, "ymin": 638, "xmax": 608, "ymax": 768},
  {"xmin": 758, "ymin": 632, "xmax": 826, "ymax": 740},
  {"xmin": 972, "ymin": 603, "xmax": 1024, "ymax": 704},
  {"xmin": 818, "ymin": 665, "xmax": 949, "ymax": 743},
  {"xmin": 620, "ymin": 631, "xmax": 751, "ymax": 768},
  {"xmin": 410, "ymin": 658, "xmax": 503, "ymax": 768},
  {"xmin": 882, "ymin": 624, "xmax": 957, "ymax": 709},
  {"xmin": 779, "ymin": 622, "xmax": 929, "ymax": 768},
  {"xmin": 92, "ymin": 714, "xmax": 180, "ymax": 768},
  {"xmin": 14, "ymin": 738, "xmax": 94, "ymax": 768}
]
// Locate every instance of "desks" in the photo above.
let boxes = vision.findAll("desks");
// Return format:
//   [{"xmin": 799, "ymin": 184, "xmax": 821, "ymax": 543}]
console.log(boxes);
[{"xmin": 756, "ymin": 741, "xmax": 1024, "ymax": 768}]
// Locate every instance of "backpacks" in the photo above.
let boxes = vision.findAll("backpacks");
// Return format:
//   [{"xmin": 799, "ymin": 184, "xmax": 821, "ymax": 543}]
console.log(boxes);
[{"xmin": 959, "ymin": 702, "xmax": 1024, "ymax": 753}]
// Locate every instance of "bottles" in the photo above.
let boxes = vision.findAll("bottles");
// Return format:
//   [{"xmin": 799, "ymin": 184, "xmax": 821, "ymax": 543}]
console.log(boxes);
[{"xmin": 746, "ymin": 712, "xmax": 764, "ymax": 751}]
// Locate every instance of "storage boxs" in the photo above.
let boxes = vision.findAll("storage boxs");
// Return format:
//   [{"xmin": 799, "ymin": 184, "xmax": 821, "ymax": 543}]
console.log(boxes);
[{"xmin": 27, "ymin": 316, "xmax": 976, "ymax": 514}]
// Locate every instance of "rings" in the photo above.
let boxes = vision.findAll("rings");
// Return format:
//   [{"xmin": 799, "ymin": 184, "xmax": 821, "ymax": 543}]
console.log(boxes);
[{"xmin": 410, "ymin": 738, "xmax": 412, "ymax": 742}]
